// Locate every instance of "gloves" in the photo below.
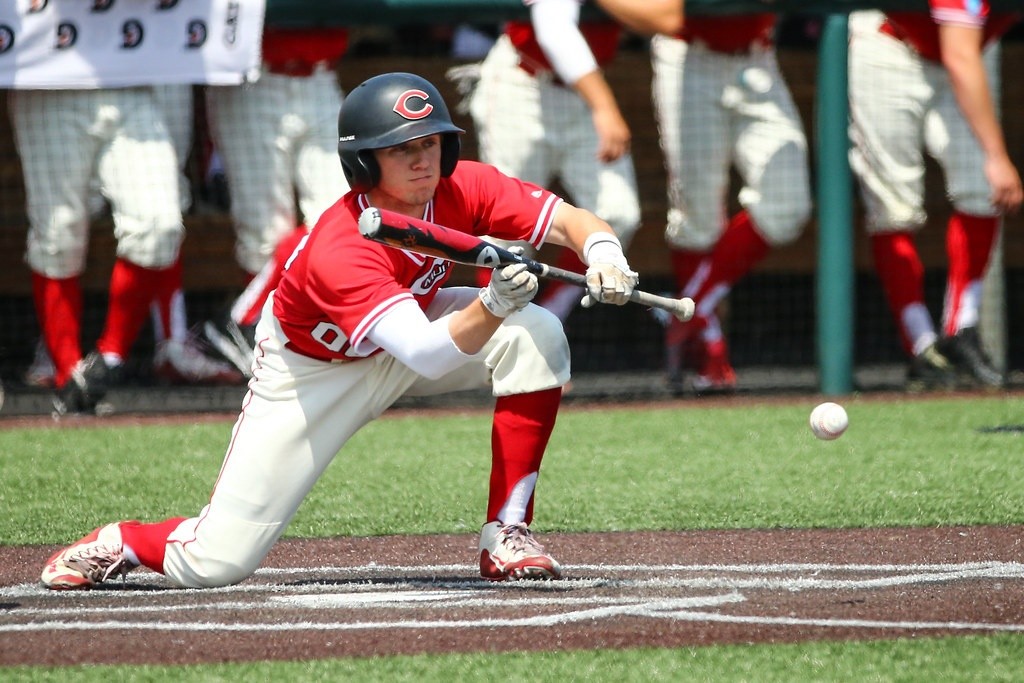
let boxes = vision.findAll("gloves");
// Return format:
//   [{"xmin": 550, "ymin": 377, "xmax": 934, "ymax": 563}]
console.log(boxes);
[
  {"xmin": 478, "ymin": 246, "xmax": 538, "ymax": 317},
  {"xmin": 579, "ymin": 241, "xmax": 638, "ymax": 309}
]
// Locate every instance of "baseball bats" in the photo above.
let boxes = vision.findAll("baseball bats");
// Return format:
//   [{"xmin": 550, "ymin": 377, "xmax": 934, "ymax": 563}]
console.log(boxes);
[{"xmin": 356, "ymin": 206, "xmax": 697, "ymax": 325}]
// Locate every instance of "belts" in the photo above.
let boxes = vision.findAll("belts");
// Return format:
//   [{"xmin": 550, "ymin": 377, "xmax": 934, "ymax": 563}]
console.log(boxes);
[{"xmin": 284, "ymin": 341, "xmax": 333, "ymax": 363}]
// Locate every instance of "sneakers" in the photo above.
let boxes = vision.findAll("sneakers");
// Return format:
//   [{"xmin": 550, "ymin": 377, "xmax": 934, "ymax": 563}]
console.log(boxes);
[
  {"xmin": 479, "ymin": 520, "xmax": 561, "ymax": 578},
  {"xmin": 51, "ymin": 351, "xmax": 129, "ymax": 419},
  {"xmin": 154, "ymin": 342, "xmax": 242, "ymax": 382},
  {"xmin": 902, "ymin": 329, "xmax": 1005, "ymax": 394},
  {"xmin": 695, "ymin": 359, "xmax": 738, "ymax": 396},
  {"xmin": 39, "ymin": 520, "xmax": 140, "ymax": 592}
]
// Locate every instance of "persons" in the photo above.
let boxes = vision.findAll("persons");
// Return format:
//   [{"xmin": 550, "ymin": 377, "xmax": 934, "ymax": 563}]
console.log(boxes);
[
  {"xmin": 848, "ymin": 0, "xmax": 1024, "ymax": 394},
  {"xmin": 42, "ymin": 72, "xmax": 639, "ymax": 591},
  {"xmin": 7, "ymin": 88, "xmax": 241, "ymax": 415},
  {"xmin": 204, "ymin": 24, "xmax": 352, "ymax": 342},
  {"xmin": 650, "ymin": 13, "xmax": 813, "ymax": 388},
  {"xmin": 469, "ymin": 0, "xmax": 685, "ymax": 324}
]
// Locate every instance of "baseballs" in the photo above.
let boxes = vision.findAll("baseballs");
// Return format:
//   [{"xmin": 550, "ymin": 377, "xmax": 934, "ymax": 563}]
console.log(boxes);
[{"xmin": 808, "ymin": 401, "xmax": 851, "ymax": 441}]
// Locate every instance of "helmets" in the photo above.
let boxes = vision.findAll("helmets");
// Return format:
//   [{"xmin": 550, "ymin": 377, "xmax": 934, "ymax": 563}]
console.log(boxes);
[{"xmin": 338, "ymin": 72, "xmax": 465, "ymax": 189}]
